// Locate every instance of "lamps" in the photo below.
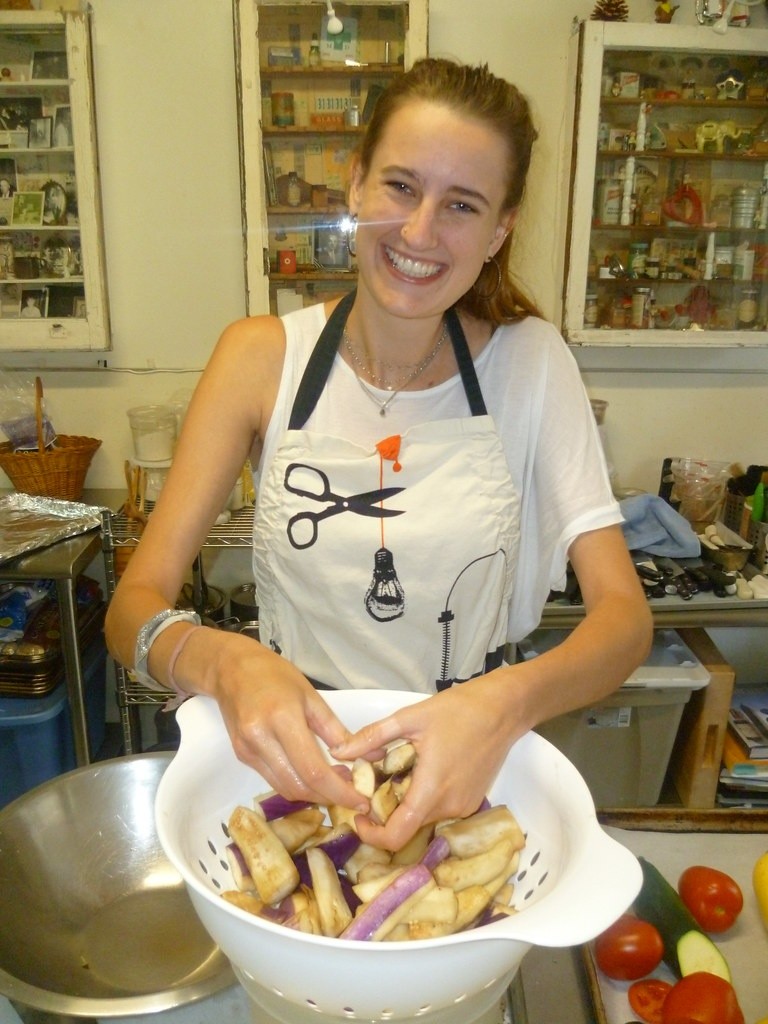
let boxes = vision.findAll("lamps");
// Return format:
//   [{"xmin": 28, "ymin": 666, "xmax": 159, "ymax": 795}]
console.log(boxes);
[{"xmin": 323, "ymin": 0, "xmax": 344, "ymax": 34}]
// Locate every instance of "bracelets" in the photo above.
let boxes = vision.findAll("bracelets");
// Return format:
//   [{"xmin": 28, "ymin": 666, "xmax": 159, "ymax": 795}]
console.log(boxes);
[{"xmin": 134, "ymin": 609, "xmax": 200, "ymax": 692}]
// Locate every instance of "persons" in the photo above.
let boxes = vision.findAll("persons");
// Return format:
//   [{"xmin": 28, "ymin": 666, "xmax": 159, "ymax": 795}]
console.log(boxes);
[
  {"xmin": 165, "ymin": 625, "xmax": 211, "ymax": 701},
  {"xmin": 22, "ymin": 294, "xmax": 42, "ymax": 318},
  {"xmin": 101, "ymin": 57, "xmax": 654, "ymax": 848}
]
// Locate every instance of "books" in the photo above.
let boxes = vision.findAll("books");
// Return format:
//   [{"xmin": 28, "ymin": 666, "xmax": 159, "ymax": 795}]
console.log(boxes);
[{"xmin": 715, "ymin": 701, "xmax": 768, "ymax": 808}]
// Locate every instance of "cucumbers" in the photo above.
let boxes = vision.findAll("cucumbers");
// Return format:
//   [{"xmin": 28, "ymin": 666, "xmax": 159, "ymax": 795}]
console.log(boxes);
[{"xmin": 631, "ymin": 855, "xmax": 730, "ymax": 983}]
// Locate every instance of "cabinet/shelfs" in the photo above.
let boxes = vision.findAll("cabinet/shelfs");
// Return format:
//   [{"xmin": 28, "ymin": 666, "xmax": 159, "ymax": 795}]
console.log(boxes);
[
  {"xmin": 1, "ymin": 0, "xmax": 115, "ymax": 356},
  {"xmin": 100, "ymin": 499, "xmax": 261, "ymax": 756},
  {"xmin": 236, "ymin": 1, "xmax": 431, "ymax": 318},
  {"xmin": 564, "ymin": 20, "xmax": 767, "ymax": 349}
]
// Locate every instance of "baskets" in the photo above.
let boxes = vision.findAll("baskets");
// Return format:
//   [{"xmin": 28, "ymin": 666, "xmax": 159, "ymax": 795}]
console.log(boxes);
[{"xmin": 1, "ymin": 375, "xmax": 103, "ymax": 506}]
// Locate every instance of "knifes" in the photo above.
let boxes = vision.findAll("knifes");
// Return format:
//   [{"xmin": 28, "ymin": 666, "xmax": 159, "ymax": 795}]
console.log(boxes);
[{"xmin": 634, "ymin": 557, "xmax": 755, "ymax": 600}]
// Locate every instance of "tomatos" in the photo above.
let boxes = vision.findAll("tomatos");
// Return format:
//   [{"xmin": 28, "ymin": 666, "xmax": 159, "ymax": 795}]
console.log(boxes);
[{"xmin": 593, "ymin": 866, "xmax": 746, "ymax": 1024}]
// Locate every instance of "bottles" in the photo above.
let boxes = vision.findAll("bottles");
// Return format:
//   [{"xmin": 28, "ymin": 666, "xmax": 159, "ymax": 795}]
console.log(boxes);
[
  {"xmin": 308, "ymin": 34, "xmax": 322, "ymax": 68},
  {"xmin": 311, "ymin": 184, "xmax": 329, "ymax": 208},
  {"xmin": 631, "ymin": 287, "xmax": 651, "ymax": 329},
  {"xmin": 584, "ymin": 294, "xmax": 601, "ymax": 329},
  {"xmin": 639, "ymin": 193, "xmax": 662, "ymax": 227},
  {"xmin": 708, "ymin": 186, "xmax": 762, "ymax": 229},
  {"xmin": 626, "ymin": 242, "xmax": 683, "ymax": 281},
  {"xmin": 287, "ymin": 173, "xmax": 301, "ymax": 207},
  {"xmin": 735, "ymin": 288, "xmax": 759, "ymax": 332}
]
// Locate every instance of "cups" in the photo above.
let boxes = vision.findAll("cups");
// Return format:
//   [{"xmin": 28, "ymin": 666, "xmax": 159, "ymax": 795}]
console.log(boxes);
[
  {"xmin": 127, "ymin": 406, "xmax": 178, "ymax": 462},
  {"xmin": 271, "ymin": 92, "xmax": 295, "ymax": 126}
]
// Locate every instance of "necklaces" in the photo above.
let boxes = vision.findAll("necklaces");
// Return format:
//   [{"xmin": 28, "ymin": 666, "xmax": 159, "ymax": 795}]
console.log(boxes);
[{"xmin": 340, "ymin": 321, "xmax": 450, "ymax": 415}]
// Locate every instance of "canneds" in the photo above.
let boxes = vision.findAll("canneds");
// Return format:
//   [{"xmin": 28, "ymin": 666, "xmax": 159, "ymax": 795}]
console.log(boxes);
[
  {"xmin": 272, "ymin": 92, "xmax": 295, "ymax": 126},
  {"xmin": 626, "ymin": 242, "xmax": 661, "ymax": 280},
  {"xmin": 630, "ymin": 287, "xmax": 652, "ymax": 329},
  {"xmin": 594, "ymin": 176, "xmax": 620, "ymax": 227},
  {"xmin": 736, "ymin": 288, "xmax": 759, "ymax": 331},
  {"xmin": 583, "ymin": 294, "xmax": 599, "ymax": 327}
]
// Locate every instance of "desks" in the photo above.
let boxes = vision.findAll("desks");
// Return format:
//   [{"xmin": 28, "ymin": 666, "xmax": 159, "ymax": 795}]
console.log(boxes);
[{"xmin": 0, "ymin": 487, "xmax": 130, "ymax": 767}]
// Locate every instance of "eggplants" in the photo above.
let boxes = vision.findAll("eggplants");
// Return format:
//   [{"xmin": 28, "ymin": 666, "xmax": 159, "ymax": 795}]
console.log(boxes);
[{"xmin": 225, "ymin": 744, "xmax": 528, "ymax": 944}]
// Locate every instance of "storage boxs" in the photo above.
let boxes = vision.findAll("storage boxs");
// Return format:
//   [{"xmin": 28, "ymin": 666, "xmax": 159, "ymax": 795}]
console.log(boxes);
[
  {"xmin": 0, "ymin": 646, "xmax": 110, "ymax": 806},
  {"xmin": 521, "ymin": 627, "xmax": 710, "ymax": 809},
  {"xmin": 668, "ymin": 630, "xmax": 735, "ymax": 807}
]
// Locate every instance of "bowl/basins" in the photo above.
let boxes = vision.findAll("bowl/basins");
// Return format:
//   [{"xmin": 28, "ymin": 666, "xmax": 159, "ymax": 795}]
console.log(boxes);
[{"xmin": 0, "ymin": 749, "xmax": 238, "ymax": 1020}]
